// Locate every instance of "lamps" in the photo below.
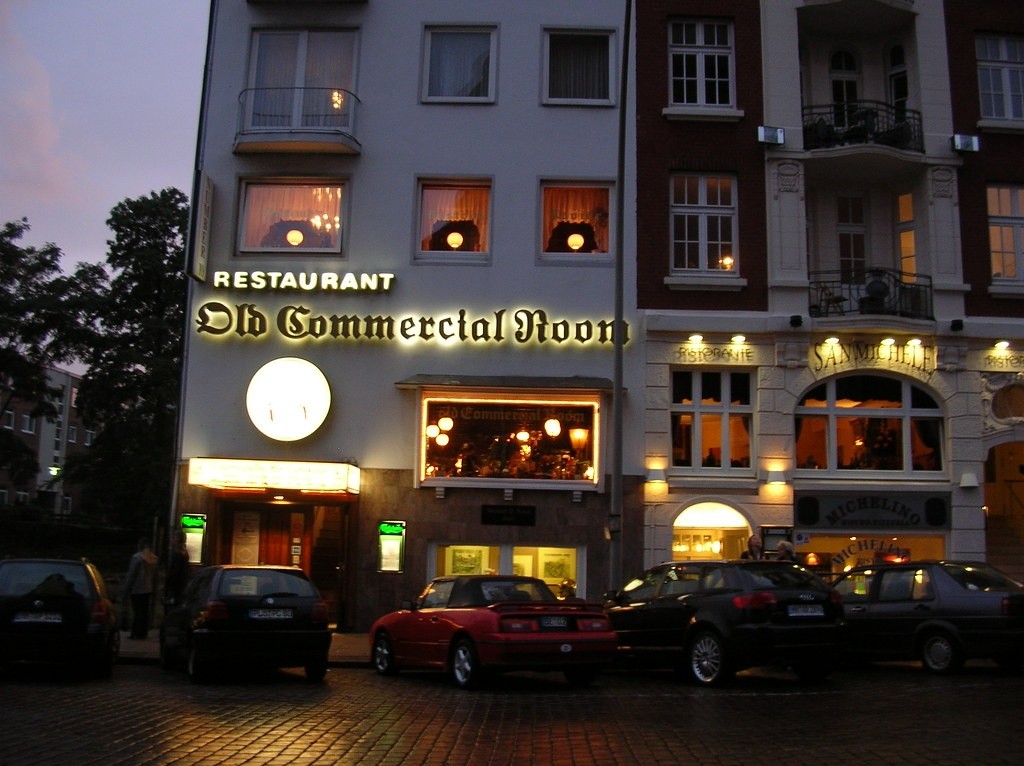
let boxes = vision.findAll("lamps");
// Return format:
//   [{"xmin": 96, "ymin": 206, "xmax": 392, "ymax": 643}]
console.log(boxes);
[
  {"xmin": 566, "ymin": 234, "xmax": 585, "ymax": 252},
  {"xmin": 959, "ymin": 473, "xmax": 980, "ymax": 488},
  {"xmin": 447, "ymin": 231, "xmax": 464, "ymax": 250},
  {"xmin": 242, "ymin": 358, "xmax": 333, "ymax": 443},
  {"xmin": 286, "ymin": 229, "xmax": 304, "ymax": 246},
  {"xmin": 950, "ymin": 134, "xmax": 979, "ymax": 155},
  {"xmin": 767, "ymin": 470, "xmax": 787, "ymax": 485},
  {"xmin": 646, "ymin": 468, "xmax": 667, "ymax": 483},
  {"xmin": 753, "ymin": 125, "xmax": 786, "ymax": 147}
]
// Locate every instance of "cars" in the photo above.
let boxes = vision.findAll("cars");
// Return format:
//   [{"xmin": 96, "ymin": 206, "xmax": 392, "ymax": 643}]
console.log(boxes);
[
  {"xmin": 159, "ymin": 564, "xmax": 335, "ymax": 682},
  {"xmin": 834, "ymin": 559, "xmax": 1024, "ymax": 674},
  {"xmin": 606, "ymin": 558, "xmax": 852, "ymax": 684},
  {"xmin": 368, "ymin": 572, "xmax": 619, "ymax": 691}
]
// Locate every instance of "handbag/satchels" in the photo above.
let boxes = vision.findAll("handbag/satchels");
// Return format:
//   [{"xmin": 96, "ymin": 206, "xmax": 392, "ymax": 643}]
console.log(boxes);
[{"xmin": 119, "ymin": 605, "xmax": 130, "ymax": 631}]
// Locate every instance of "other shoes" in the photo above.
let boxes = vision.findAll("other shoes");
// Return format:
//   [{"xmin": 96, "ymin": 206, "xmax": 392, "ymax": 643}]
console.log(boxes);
[{"xmin": 127, "ymin": 634, "xmax": 140, "ymax": 639}]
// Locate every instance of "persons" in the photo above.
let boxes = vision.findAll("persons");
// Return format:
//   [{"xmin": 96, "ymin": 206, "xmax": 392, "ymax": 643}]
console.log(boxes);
[
  {"xmin": 776, "ymin": 541, "xmax": 797, "ymax": 560},
  {"xmin": 123, "ymin": 537, "xmax": 158, "ymax": 640},
  {"xmin": 160, "ymin": 531, "xmax": 189, "ymax": 601},
  {"xmin": 859, "ymin": 456, "xmax": 873, "ymax": 470},
  {"xmin": 741, "ymin": 534, "xmax": 766, "ymax": 559}
]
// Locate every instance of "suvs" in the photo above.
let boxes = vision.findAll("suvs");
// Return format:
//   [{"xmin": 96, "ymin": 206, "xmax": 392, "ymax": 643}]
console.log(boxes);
[{"xmin": 0, "ymin": 554, "xmax": 120, "ymax": 675}]
